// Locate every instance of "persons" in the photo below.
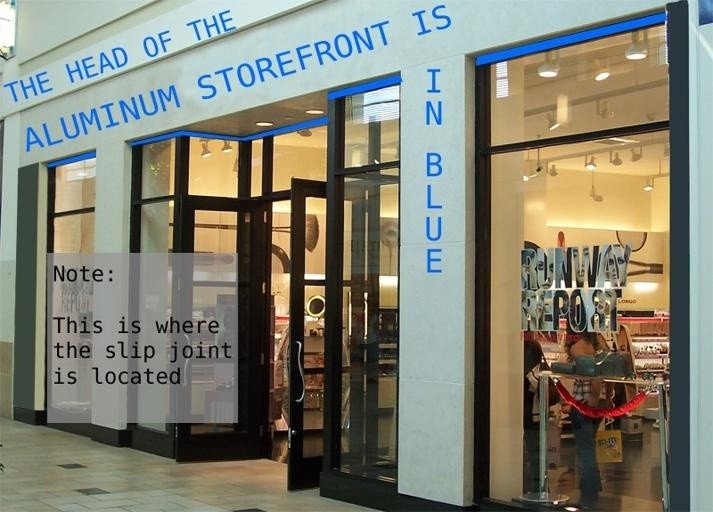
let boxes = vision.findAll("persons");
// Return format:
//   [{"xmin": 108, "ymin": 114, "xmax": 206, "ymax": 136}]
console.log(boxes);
[
  {"xmin": 521, "ymin": 244, "xmax": 631, "ymax": 329},
  {"xmin": 564, "ymin": 332, "xmax": 615, "ymax": 506},
  {"xmin": 524, "ymin": 341, "xmax": 542, "ymax": 492}
]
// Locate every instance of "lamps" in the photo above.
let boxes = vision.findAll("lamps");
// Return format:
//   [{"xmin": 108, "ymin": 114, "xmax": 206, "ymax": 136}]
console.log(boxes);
[
  {"xmin": 200, "ymin": 139, "xmax": 212, "ymax": 158},
  {"xmin": 221, "ymin": 140, "xmax": 232, "ymax": 153},
  {"xmin": 537, "ymin": 50, "xmax": 560, "ymax": 77},
  {"xmin": 523, "ymin": 96, "xmax": 669, "ymax": 202},
  {"xmin": 625, "ymin": 30, "xmax": 649, "ymax": 60},
  {"xmin": 592, "ymin": 58, "xmax": 611, "ymax": 82},
  {"xmin": 297, "ymin": 129, "xmax": 312, "ymax": 136}
]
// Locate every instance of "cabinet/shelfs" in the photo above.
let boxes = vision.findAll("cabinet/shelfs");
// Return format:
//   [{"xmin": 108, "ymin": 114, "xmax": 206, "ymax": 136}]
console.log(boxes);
[
  {"xmin": 525, "ymin": 317, "xmax": 670, "ymax": 419},
  {"xmin": 275, "ymin": 315, "xmax": 350, "ymax": 463},
  {"xmin": 378, "ymin": 306, "xmax": 398, "ymax": 415}
]
